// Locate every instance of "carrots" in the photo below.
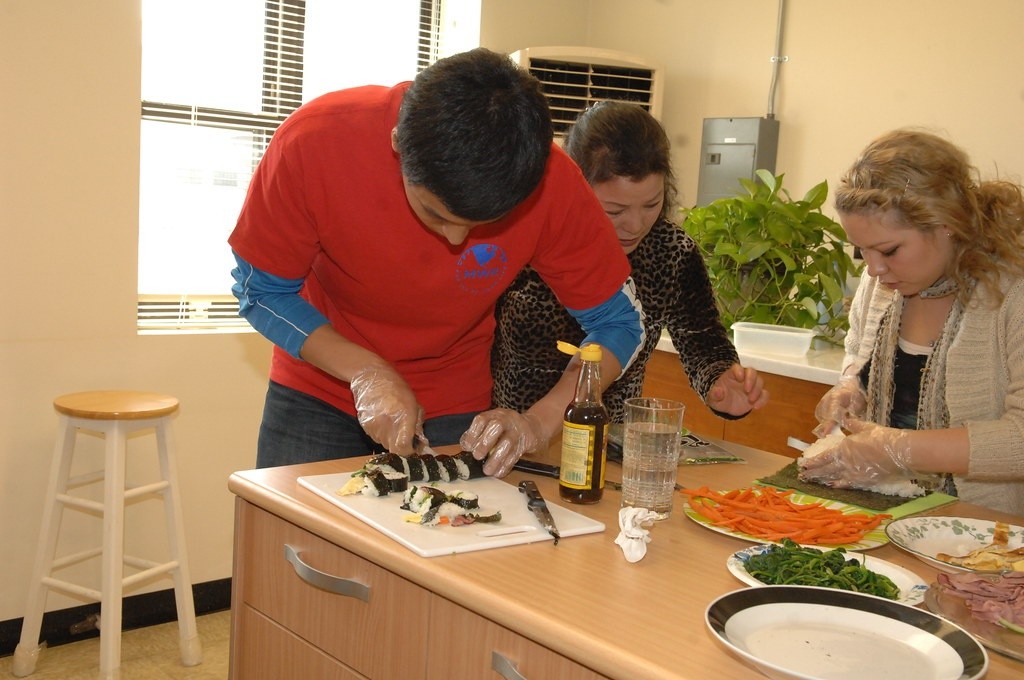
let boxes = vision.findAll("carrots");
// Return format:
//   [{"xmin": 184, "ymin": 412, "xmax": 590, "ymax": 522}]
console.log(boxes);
[{"xmin": 680, "ymin": 485, "xmax": 891, "ymax": 546}]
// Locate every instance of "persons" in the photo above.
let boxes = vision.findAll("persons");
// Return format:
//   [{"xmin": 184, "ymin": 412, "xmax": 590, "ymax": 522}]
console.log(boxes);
[
  {"xmin": 226, "ymin": 45, "xmax": 647, "ymax": 481},
  {"xmin": 491, "ymin": 100, "xmax": 772, "ymax": 424},
  {"xmin": 795, "ymin": 126, "xmax": 1024, "ymax": 518}
]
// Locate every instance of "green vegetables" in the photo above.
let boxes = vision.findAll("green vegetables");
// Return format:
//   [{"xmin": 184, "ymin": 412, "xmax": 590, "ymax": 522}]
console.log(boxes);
[{"xmin": 744, "ymin": 538, "xmax": 900, "ymax": 599}]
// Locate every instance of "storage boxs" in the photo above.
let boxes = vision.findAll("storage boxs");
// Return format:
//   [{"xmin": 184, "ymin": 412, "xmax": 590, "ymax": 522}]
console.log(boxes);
[{"xmin": 731, "ymin": 322, "xmax": 816, "ymax": 356}]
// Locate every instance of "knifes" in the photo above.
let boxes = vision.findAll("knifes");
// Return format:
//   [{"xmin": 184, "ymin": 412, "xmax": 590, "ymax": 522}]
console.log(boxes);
[
  {"xmin": 518, "ymin": 481, "xmax": 559, "ymax": 538},
  {"xmin": 513, "ymin": 458, "xmax": 622, "ymax": 491},
  {"xmin": 411, "ymin": 436, "xmax": 438, "ymax": 458}
]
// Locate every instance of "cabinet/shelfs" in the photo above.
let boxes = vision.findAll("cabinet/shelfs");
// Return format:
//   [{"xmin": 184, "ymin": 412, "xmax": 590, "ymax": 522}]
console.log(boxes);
[{"xmin": 229, "ymin": 423, "xmax": 1024, "ymax": 680}]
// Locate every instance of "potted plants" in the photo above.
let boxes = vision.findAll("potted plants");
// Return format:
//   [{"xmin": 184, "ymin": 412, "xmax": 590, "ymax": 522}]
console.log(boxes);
[{"xmin": 677, "ymin": 169, "xmax": 865, "ymax": 348}]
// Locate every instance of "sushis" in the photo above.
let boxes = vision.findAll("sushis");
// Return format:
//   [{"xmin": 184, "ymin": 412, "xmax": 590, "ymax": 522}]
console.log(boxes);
[{"xmin": 339, "ymin": 447, "xmax": 503, "ymax": 527}]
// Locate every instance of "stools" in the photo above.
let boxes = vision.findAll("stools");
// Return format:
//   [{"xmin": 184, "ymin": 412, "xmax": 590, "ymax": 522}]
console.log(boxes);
[{"xmin": 11, "ymin": 390, "xmax": 202, "ymax": 680}]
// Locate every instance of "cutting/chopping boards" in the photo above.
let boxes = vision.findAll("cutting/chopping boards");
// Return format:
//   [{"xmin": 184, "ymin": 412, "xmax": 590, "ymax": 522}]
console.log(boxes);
[{"xmin": 296, "ymin": 472, "xmax": 605, "ymax": 558}]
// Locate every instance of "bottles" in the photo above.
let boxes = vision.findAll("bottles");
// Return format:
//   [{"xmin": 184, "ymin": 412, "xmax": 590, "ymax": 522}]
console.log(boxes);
[{"xmin": 557, "ymin": 340, "xmax": 606, "ymax": 504}]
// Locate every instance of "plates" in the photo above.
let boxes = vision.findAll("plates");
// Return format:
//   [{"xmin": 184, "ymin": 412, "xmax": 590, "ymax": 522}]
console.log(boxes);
[
  {"xmin": 685, "ymin": 491, "xmax": 890, "ymax": 550},
  {"xmin": 885, "ymin": 516, "xmax": 1024, "ymax": 574},
  {"xmin": 727, "ymin": 545, "xmax": 929, "ymax": 606},
  {"xmin": 705, "ymin": 585, "xmax": 989, "ymax": 680},
  {"xmin": 925, "ymin": 585, "xmax": 1024, "ymax": 661}
]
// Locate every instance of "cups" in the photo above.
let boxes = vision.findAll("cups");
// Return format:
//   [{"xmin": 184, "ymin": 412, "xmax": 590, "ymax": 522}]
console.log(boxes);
[{"xmin": 623, "ymin": 397, "xmax": 685, "ymax": 512}]
